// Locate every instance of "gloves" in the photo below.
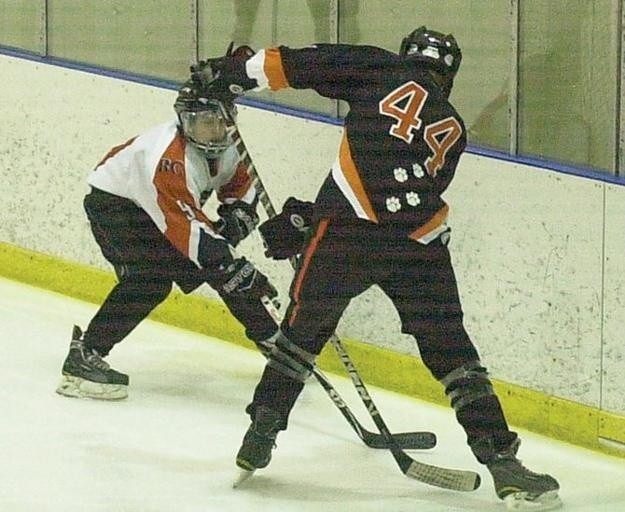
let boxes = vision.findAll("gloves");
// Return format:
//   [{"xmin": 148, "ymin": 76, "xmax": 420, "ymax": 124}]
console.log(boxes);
[
  {"xmin": 211, "ymin": 200, "xmax": 259, "ymax": 248},
  {"xmin": 259, "ymin": 196, "xmax": 315, "ymax": 261},
  {"xmin": 189, "ymin": 41, "xmax": 260, "ymax": 104},
  {"xmin": 217, "ymin": 256, "xmax": 281, "ymax": 314}
]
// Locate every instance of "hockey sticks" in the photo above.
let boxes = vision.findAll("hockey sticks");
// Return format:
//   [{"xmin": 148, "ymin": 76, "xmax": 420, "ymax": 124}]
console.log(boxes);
[{"xmin": 203, "ymin": 66, "xmax": 480, "ymax": 491}]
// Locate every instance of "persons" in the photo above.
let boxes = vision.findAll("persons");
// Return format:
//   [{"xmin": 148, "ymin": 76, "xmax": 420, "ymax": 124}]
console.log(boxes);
[
  {"xmin": 56, "ymin": 74, "xmax": 312, "ymax": 401},
  {"xmin": 189, "ymin": 25, "xmax": 561, "ymax": 511}
]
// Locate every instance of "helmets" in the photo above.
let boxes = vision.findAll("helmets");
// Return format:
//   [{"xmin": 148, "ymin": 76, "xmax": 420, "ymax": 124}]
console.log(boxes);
[
  {"xmin": 174, "ymin": 77, "xmax": 239, "ymax": 160},
  {"xmin": 399, "ymin": 24, "xmax": 461, "ymax": 99}
]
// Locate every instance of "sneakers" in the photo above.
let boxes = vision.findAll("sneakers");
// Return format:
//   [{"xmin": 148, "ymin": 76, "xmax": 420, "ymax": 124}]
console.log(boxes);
[
  {"xmin": 236, "ymin": 405, "xmax": 285, "ymax": 471},
  {"xmin": 487, "ymin": 451, "xmax": 558, "ymax": 498},
  {"xmin": 61, "ymin": 325, "xmax": 130, "ymax": 384}
]
